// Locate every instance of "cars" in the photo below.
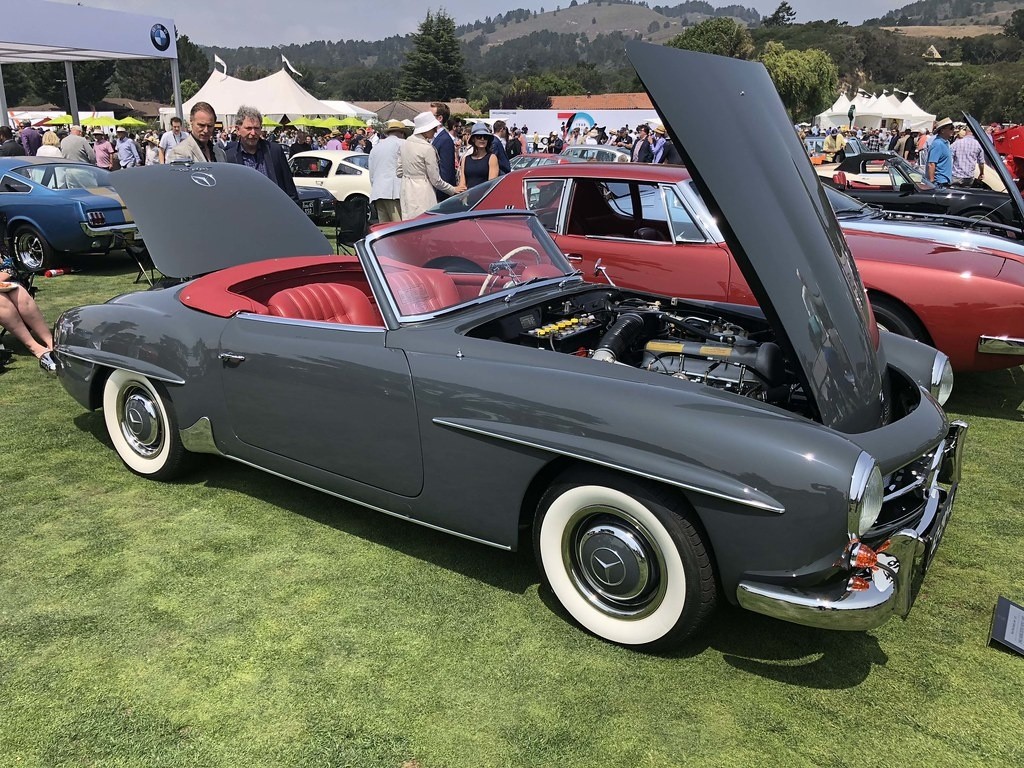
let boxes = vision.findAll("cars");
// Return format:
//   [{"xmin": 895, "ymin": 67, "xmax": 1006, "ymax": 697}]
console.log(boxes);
[
  {"xmin": 293, "ymin": 186, "xmax": 338, "ymax": 223},
  {"xmin": 802, "ymin": 136, "xmax": 869, "ymax": 162},
  {"xmin": 368, "ymin": 161, "xmax": 1024, "ymax": 388},
  {"xmin": 0, "ymin": 156, "xmax": 143, "ymax": 275},
  {"xmin": 287, "ymin": 150, "xmax": 378, "ymax": 223},
  {"xmin": 823, "ymin": 110, "xmax": 1024, "ymax": 240},
  {"xmin": 504, "ymin": 153, "xmax": 588, "ymax": 200},
  {"xmin": 46, "ymin": 45, "xmax": 966, "ymax": 659},
  {"xmin": 556, "ymin": 144, "xmax": 633, "ymax": 163}
]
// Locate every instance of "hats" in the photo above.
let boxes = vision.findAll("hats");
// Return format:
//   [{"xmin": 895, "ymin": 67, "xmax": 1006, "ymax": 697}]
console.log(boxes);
[
  {"xmin": 356, "ymin": 135, "xmax": 366, "ymax": 141},
  {"xmin": 551, "ymin": 131, "xmax": 558, "ymax": 136},
  {"xmin": 383, "ymin": 121, "xmax": 410, "ymax": 136},
  {"xmin": 330, "ymin": 130, "xmax": 341, "ymax": 138},
  {"xmin": 413, "ymin": 111, "xmax": 441, "ymax": 134},
  {"xmin": 653, "ymin": 124, "xmax": 665, "ymax": 134},
  {"xmin": 141, "ymin": 131, "xmax": 145, "ymax": 133},
  {"xmin": 619, "ymin": 127, "xmax": 626, "ymax": 132},
  {"xmin": 468, "ymin": 123, "xmax": 494, "ymax": 145},
  {"xmin": 932, "ymin": 117, "xmax": 952, "ymax": 135},
  {"xmin": 71, "ymin": 125, "xmax": 82, "ymax": 132},
  {"xmin": 116, "ymin": 127, "xmax": 127, "ymax": 133},
  {"xmin": 344, "ymin": 134, "xmax": 351, "ymax": 138},
  {"xmin": 910, "ymin": 128, "xmax": 921, "ymax": 133},
  {"xmin": 144, "ymin": 136, "xmax": 157, "ymax": 145},
  {"xmin": 829, "ymin": 128, "xmax": 838, "ymax": 134},
  {"xmin": 91, "ymin": 129, "xmax": 104, "ymax": 135}
]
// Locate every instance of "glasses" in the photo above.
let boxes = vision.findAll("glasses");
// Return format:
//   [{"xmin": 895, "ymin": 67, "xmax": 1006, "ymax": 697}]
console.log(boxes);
[
  {"xmin": 944, "ymin": 125, "xmax": 954, "ymax": 130},
  {"xmin": 476, "ymin": 135, "xmax": 488, "ymax": 140}
]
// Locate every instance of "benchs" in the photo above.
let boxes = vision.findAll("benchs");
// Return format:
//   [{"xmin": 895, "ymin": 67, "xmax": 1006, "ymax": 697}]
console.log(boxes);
[{"xmin": 529, "ymin": 208, "xmax": 618, "ymax": 233}]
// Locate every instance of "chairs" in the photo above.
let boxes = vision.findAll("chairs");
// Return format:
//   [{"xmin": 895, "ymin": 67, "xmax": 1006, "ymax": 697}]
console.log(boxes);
[
  {"xmin": 0, "ymin": 243, "xmax": 38, "ymax": 337},
  {"xmin": 268, "ymin": 282, "xmax": 375, "ymax": 330},
  {"xmin": 833, "ymin": 171, "xmax": 847, "ymax": 189},
  {"xmin": 111, "ymin": 228, "xmax": 155, "ymax": 288},
  {"xmin": 383, "ymin": 270, "xmax": 459, "ymax": 316},
  {"xmin": 334, "ymin": 192, "xmax": 372, "ymax": 256},
  {"xmin": 7, "ymin": 169, "xmax": 32, "ymax": 191}
]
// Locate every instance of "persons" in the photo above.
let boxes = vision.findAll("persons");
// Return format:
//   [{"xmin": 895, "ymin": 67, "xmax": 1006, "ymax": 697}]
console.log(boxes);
[
  {"xmin": 0, "ymin": 245, "xmax": 53, "ymax": 359},
  {"xmin": 370, "ymin": 101, "xmax": 531, "ymax": 223},
  {"xmin": 0, "ymin": 119, "xmax": 160, "ymax": 188},
  {"xmin": 167, "ymin": 102, "xmax": 227, "ymax": 163},
  {"xmin": 532, "ymin": 132, "xmax": 538, "ymax": 152},
  {"xmin": 349, "ymin": 135, "xmax": 367, "ymax": 168},
  {"xmin": 126, "ymin": 335, "xmax": 147, "ymax": 360},
  {"xmin": 88, "ymin": 319, "xmax": 108, "ymax": 351},
  {"xmin": 546, "ymin": 122, "xmax": 667, "ymax": 163},
  {"xmin": 158, "ymin": 117, "xmax": 189, "ymax": 166},
  {"xmin": 949, "ymin": 127, "xmax": 984, "ymax": 185},
  {"xmin": 327, "ymin": 130, "xmax": 343, "ymax": 150},
  {"xmin": 260, "ymin": 128, "xmax": 379, "ymax": 171},
  {"xmin": 925, "ymin": 116, "xmax": 954, "ymax": 188},
  {"xmin": 357, "ymin": 129, "xmax": 373, "ymax": 154},
  {"xmin": 224, "ymin": 106, "xmax": 299, "ymax": 204},
  {"xmin": 61, "ymin": 316, "xmax": 77, "ymax": 344},
  {"xmin": 798, "ymin": 119, "xmax": 1001, "ymax": 165}
]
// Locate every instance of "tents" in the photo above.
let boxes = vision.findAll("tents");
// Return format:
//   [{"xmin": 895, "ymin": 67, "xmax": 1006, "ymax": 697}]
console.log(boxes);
[
  {"xmin": 157, "ymin": 68, "xmax": 341, "ymax": 131},
  {"xmin": 815, "ymin": 94, "xmax": 936, "ymax": 133},
  {"xmin": 320, "ymin": 100, "xmax": 378, "ymax": 122}
]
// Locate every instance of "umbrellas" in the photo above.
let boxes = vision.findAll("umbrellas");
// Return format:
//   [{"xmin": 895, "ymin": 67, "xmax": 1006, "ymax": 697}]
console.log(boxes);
[
  {"xmin": 10, "ymin": 112, "xmax": 148, "ymax": 127},
  {"xmin": 262, "ymin": 116, "xmax": 367, "ymax": 128}
]
